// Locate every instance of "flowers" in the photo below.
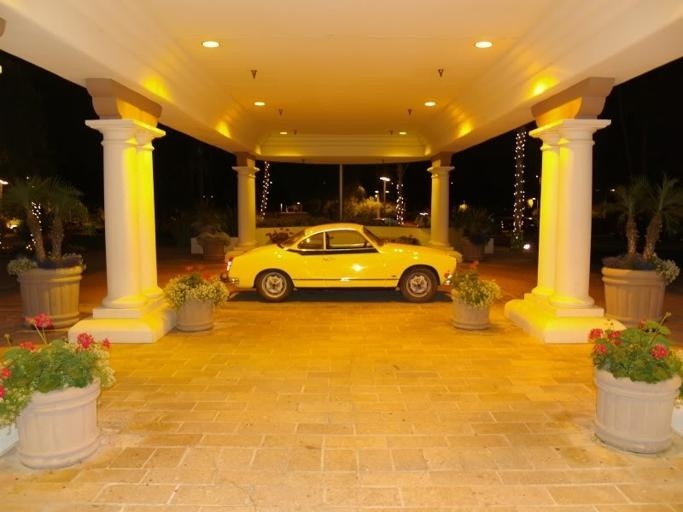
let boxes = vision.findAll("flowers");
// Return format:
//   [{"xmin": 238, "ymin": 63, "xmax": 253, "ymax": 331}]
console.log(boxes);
[
  {"xmin": 588, "ymin": 310, "xmax": 683, "ymax": 403},
  {"xmin": 161, "ymin": 270, "xmax": 231, "ymax": 317},
  {"xmin": 0, "ymin": 313, "xmax": 118, "ymax": 436}
]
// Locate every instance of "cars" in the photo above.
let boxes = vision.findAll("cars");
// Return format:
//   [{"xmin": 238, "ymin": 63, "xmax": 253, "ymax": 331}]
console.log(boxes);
[{"xmin": 226, "ymin": 223, "xmax": 457, "ymax": 303}]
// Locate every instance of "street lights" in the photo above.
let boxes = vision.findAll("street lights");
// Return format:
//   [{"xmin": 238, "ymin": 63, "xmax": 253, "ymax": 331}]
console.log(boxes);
[{"xmin": 380, "ymin": 177, "xmax": 391, "ymax": 217}]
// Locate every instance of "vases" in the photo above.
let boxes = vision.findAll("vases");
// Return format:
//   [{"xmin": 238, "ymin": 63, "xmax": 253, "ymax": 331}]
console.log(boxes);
[
  {"xmin": 591, "ymin": 368, "xmax": 683, "ymax": 454},
  {"xmin": 13, "ymin": 375, "xmax": 102, "ymax": 470},
  {"xmin": 173, "ymin": 298, "xmax": 216, "ymax": 333}
]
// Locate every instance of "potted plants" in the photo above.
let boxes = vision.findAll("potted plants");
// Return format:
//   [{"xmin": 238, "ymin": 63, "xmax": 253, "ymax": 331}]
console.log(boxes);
[
  {"xmin": 5, "ymin": 176, "xmax": 97, "ymax": 331},
  {"xmin": 195, "ymin": 231, "xmax": 232, "ymax": 262},
  {"xmin": 600, "ymin": 173, "xmax": 681, "ymax": 330},
  {"xmin": 463, "ymin": 229, "xmax": 485, "ymax": 263},
  {"xmin": 446, "ymin": 269, "xmax": 504, "ymax": 330}
]
